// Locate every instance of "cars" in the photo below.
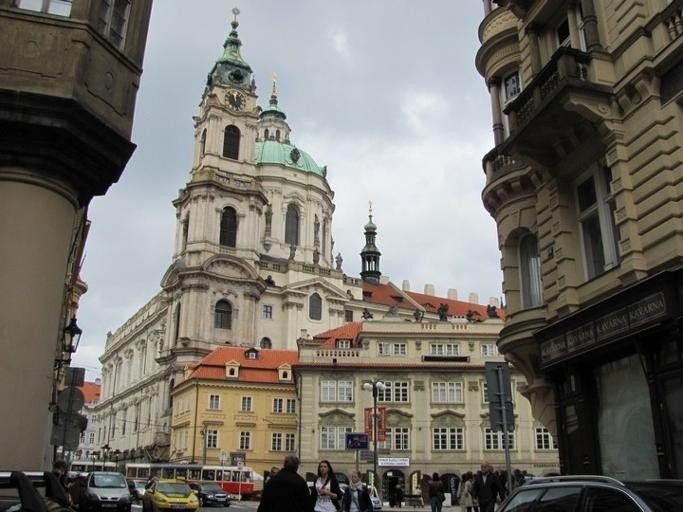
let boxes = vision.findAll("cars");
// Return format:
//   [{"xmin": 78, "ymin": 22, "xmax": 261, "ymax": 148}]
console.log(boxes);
[
  {"xmin": 305, "ymin": 481, "xmax": 382, "ymax": 511},
  {"xmin": 184, "ymin": 479, "xmax": 231, "ymax": 507},
  {"xmin": 67, "ymin": 471, "xmax": 90, "ymax": 507},
  {"xmin": 141, "ymin": 478, "xmax": 199, "ymax": 511},
  {"xmin": 125, "ymin": 478, "xmax": 147, "ymax": 499},
  {"xmin": 0, "ymin": 471, "xmax": 49, "ymax": 512},
  {"xmin": 78, "ymin": 471, "xmax": 133, "ymax": 512}
]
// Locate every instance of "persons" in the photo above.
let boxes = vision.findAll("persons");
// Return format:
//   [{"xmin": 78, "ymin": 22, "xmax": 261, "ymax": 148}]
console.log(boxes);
[
  {"xmin": 335, "ymin": 252, "xmax": 345, "ymax": 270},
  {"xmin": 256, "ymin": 455, "xmax": 525, "ymax": 511}
]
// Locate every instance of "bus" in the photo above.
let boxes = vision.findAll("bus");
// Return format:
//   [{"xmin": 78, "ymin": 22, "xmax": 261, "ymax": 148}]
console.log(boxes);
[
  {"xmin": 198, "ymin": 465, "xmax": 253, "ymax": 500},
  {"xmin": 124, "ymin": 463, "xmax": 203, "ymax": 500},
  {"xmin": 67, "ymin": 461, "xmax": 119, "ymax": 482}
]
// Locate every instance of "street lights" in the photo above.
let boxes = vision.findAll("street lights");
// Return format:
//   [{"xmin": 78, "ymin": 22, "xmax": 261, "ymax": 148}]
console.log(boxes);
[
  {"xmin": 90, "ymin": 451, "xmax": 98, "ymax": 471},
  {"xmin": 99, "ymin": 444, "xmax": 111, "ymax": 471},
  {"xmin": 113, "ymin": 448, "xmax": 121, "ymax": 471},
  {"xmin": 200, "ymin": 422, "xmax": 206, "ymax": 464},
  {"xmin": 361, "ymin": 378, "xmax": 386, "ymax": 492}
]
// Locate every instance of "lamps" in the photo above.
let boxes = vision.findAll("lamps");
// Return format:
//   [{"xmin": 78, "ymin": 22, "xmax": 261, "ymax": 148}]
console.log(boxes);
[{"xmin": 56, "ymin": 312, "xmax": 83, "ymax": 363}]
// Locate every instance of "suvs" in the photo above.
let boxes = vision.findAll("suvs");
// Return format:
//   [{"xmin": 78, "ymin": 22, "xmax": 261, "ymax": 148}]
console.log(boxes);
[{"xmin": 493, "ymin": 475, "xmax": 682, "ymax": 511}]
